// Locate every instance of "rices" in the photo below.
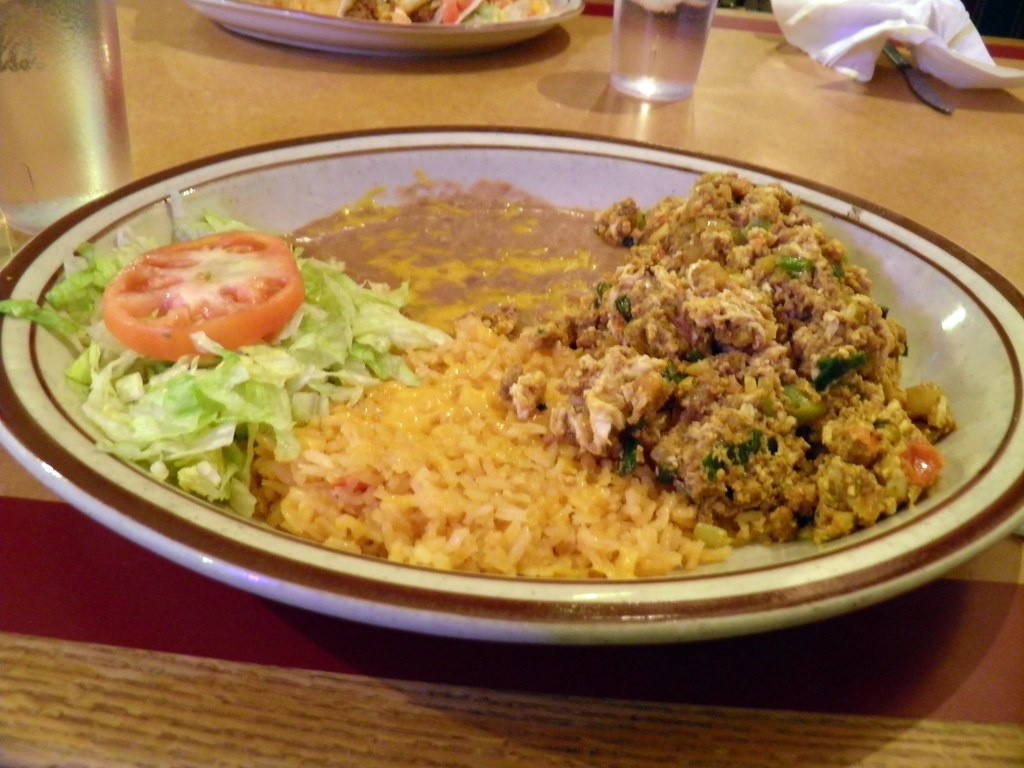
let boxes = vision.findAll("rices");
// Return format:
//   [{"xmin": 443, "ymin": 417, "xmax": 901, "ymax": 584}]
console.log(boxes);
[{"xmin": 240, "ymin": 315, "xmax": 749, "ymax": 584}]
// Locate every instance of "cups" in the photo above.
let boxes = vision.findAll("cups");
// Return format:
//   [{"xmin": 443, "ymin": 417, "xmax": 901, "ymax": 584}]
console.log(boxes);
[
  {"xmin": 1, "ymin": 0, "xmax": 135, "ymax": 233},
  {"xmin": 607, "ymin": 0, "xmax": 719, "ymax": 103}
]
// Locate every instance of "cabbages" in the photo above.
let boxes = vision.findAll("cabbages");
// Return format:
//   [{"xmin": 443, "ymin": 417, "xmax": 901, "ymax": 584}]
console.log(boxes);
[{"xmin": 0, "ymin": 183, "xmax": 457, "ymax": 518}]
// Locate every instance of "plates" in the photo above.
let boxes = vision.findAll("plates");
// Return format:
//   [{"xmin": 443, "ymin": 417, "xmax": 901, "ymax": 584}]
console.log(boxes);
[
  {"xmin": 0, "ymin": 123, "xmax": 1024, "ymax": 647},
  {"xmin": 186, "ymin": 0, "xmax": 587, "ymax": 58}
]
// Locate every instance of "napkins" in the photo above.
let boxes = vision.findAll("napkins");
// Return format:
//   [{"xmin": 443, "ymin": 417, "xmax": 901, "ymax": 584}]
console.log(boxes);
[{"xmin": 771, "ymin": 0, "xmax": 1024, "ymax": 93}]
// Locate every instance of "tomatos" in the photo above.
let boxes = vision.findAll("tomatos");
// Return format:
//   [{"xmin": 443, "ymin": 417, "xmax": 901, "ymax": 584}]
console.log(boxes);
[{"xmin": 101, "ymin": 231, "xmax": 306, "ymax": 360}]
